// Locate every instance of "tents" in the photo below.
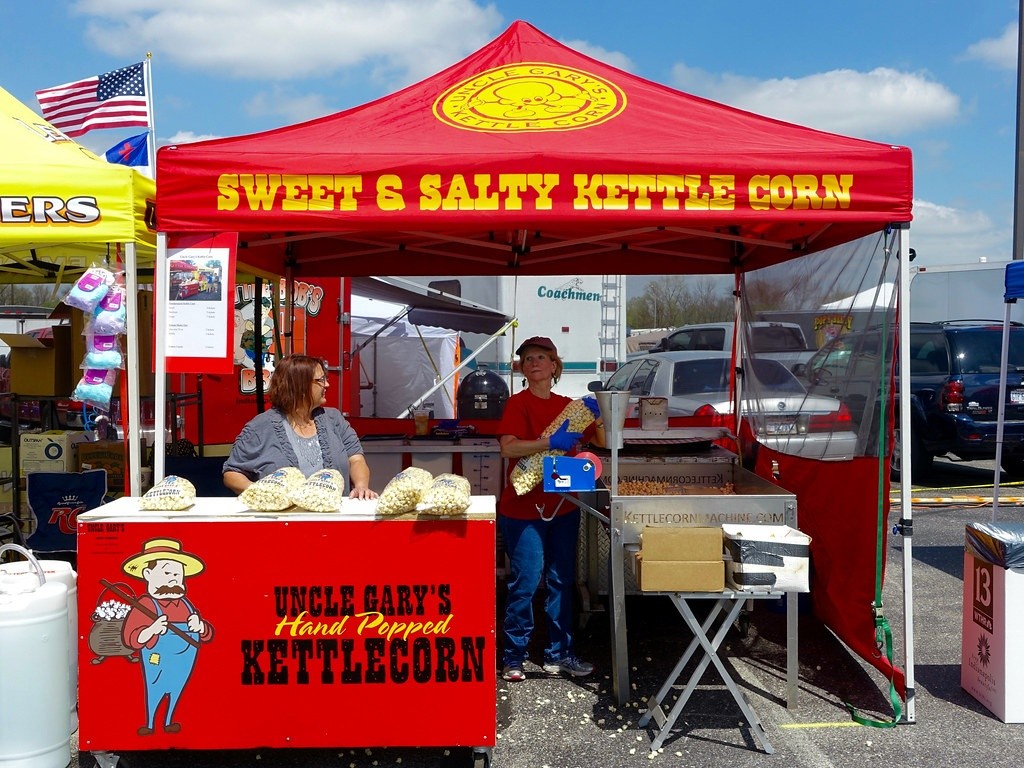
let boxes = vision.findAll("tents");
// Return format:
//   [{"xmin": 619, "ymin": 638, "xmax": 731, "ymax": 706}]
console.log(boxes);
[
  {"xmin": 169, "ymin": 260, "xmax": 197, "ymax": 284},
  {"xmin": 0, "ymin": 84, "xmax": 279, "ymax": 497},
  {"xmin": 991, "ymin": 257, "xmax": 1024, "ymax": 529},
  {"xmin": 194, "ymin": 262, "xmax": 214, "ymax": 281},
  {"xmin": 150, "ymin": 19, "xmax": 916, "ymax": 720}
]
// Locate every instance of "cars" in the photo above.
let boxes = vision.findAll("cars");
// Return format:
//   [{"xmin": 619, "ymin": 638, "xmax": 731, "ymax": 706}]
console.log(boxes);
[{"xmin": 586, "ymin": 349, "xmax": 859, "ymax": 463}]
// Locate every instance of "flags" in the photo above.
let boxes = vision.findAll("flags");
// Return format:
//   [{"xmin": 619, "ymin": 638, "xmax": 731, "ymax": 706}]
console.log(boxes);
[
  {"xmin": 99, "ymin": 131, "xmax": 150, "ymax": 167},
  {"xmin": 36, "ymin": 60, "xmax": 150, "ymax": 138}
]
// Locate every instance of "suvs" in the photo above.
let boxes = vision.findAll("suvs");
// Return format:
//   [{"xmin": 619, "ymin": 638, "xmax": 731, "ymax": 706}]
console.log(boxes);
[{"xmin": 788, "ymin": 317, "xmax": 1024, "ymax": 484}]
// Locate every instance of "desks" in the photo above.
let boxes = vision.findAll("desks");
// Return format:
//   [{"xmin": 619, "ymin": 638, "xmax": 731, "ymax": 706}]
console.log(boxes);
[
  {"xmin": 633, "ymin": 589, "xmax": 789, "ymax": 754},
  {"xmin": 76, "ymin": 494, "xmax": 498, "ymax": 768}
]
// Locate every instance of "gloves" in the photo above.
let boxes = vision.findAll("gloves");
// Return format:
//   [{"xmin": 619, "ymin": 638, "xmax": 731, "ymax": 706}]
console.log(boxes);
[
  {"xmin": 583, "ymin": 396, "xmax": 602, "ymax": 421},
  {"xmin": 548, "ymin": 419, "xmax": 584, "ymax": 450}
]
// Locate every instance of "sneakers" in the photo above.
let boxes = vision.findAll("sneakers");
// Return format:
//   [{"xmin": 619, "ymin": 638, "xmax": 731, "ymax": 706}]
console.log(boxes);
[
  {"xmin": 544, "ymin": 655, "xmax": 594, "ymax": 679},
  {"xmin": 501, "ymin": 663, "xmax": 526, "ymax": 682}
]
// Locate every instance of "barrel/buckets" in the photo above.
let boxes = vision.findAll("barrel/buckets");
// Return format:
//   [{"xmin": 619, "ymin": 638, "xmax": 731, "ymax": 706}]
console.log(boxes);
[{"xmin": 0, "ymin": 560, "xmax": 79, "ymax": 768}]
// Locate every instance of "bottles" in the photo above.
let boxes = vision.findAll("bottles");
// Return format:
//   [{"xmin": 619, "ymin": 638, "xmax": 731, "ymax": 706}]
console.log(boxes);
[{"xmin": 423, "ymin": 403, "xmax": 434, "ymax": 418}]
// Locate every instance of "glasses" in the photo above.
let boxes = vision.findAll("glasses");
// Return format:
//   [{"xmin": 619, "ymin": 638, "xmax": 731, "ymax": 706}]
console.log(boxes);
[{"xmin": 312, "ymin": 375, "xmax": 329, "ymax": 386}]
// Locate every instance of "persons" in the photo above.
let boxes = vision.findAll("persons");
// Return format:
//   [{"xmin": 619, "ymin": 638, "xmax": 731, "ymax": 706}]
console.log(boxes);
[
  {"xmin": 198, "ymin": 271, "xmax": 219, "ymax": 294},
  {"xmin": 223, "ymin": 354, "xmax": 380, "ymax": 502},
  {"xmin": 498, "ymin": 335, "xmax": 605, "ymax": 682}
]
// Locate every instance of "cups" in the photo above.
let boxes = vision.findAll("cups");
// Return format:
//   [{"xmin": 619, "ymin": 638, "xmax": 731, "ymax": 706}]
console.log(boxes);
[{"xmin": 414, "ymin": 410, "xmax": 430, "ymax": 435}]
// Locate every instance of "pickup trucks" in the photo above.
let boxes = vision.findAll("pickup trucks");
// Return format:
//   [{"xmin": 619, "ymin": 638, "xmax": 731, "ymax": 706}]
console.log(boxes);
[{"xmin": 646, "ymin": 321, "xmax": 877, "ymax": 394}]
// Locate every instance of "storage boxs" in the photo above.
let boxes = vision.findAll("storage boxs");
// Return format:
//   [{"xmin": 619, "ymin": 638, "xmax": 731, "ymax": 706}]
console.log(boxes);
[
  {"xmin": 722, "ymin": 522, "xmax": 813, "ymax": 597},
  {"xmin": 46, "ymin": 288, "xmax": 170, "ymax": 397},
  {"xmin": 17, "ymin": 429, "xmax": 95, "ymax": 485},
  {"xmin": 76, "ymin": 437, "xmax": 149, "ymax": 492},
  {"xmin": 0, "ymin": 321, "xmax": 70, "ymax": 398},
  {"xmin": 630, "ymin": 559, "xmax": 727, "ymax": 595},
  {"xmin": 639, "ymin": 522, "xmax": 725, "ymax": 560}
]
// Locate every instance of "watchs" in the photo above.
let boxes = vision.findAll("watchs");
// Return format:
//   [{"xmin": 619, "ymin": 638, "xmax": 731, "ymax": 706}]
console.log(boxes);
[{"xmin": 596, "ymin": 420, "xmax": 605, "ymax": 429}]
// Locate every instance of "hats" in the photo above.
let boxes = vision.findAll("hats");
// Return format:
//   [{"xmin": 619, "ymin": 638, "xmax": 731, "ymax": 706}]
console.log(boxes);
[{"xmin": 515, "ymin": 337, "xmax": 558, "ymax": 357}]
